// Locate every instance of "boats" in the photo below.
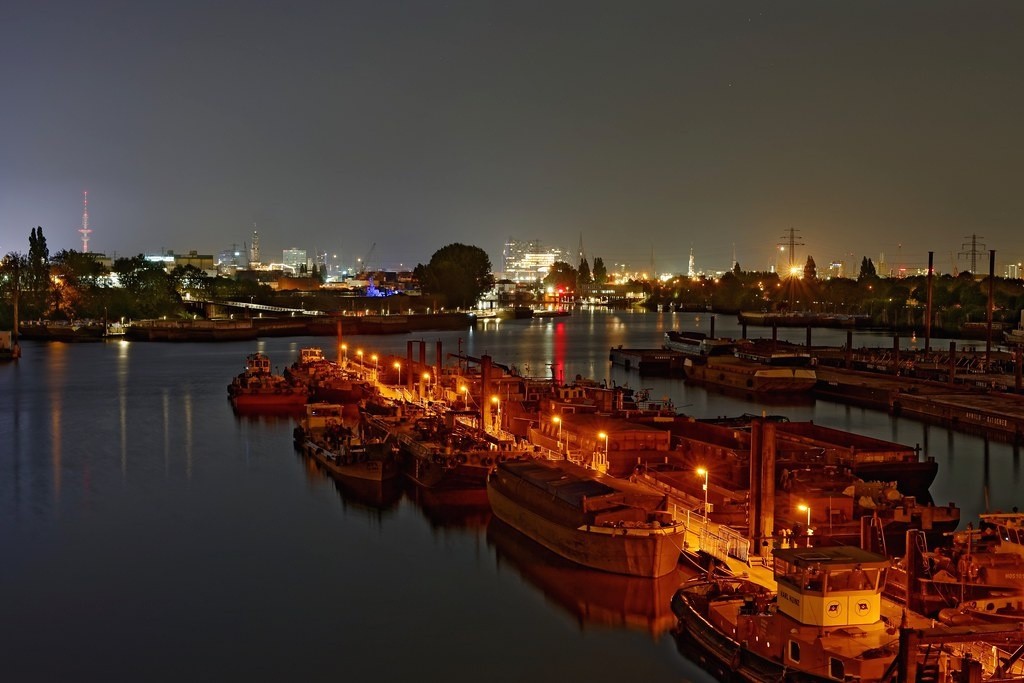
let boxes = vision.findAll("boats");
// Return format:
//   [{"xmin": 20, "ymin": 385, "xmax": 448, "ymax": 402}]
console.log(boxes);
[
  {"xmin": 774, "ymin": 462, "xmax": 959, "ymax": 555},
  {"xmin": 684, "ymin": 336, "xmax": 818, "ymax": 400},
  {"xmin": 293, "ymin": 335, "xmax": 683, "ymax": 492},
  {"xmin": 0, "ymin": 326, "xmax": 22, "ymax": 361},
  {"xmin": 486, "ymin": 452, "xmax": 686, "ymax": 580},
  {"xmin": 495, "ymin": 307, "xmax": 534, "ymax": 321},
  {"xmin": 938, "ymin": 594, "xmax": 1024, "ymax": 654},
  {"xmin": 696, "ymin": 411, "xmax": 940, "ymax": 498},
  {"xmin": 880, "ymin": 510, "xmax": 1024, "ymax": 616},
  {"xmin": 671, "ymin": 545, "xmax": 902, "ymax": 683},
  {"xmin": 226, "ymin": 345, "xmax": 373, "ymax": 420},
  {"xmin": 15, "ymin": 305, "xmax": 108, "ymax": 343}
]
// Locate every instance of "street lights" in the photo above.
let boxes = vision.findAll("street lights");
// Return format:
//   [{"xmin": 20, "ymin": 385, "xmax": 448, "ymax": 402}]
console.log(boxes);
[
  {"xmin": 491, "ymin": 396, "xmax": 500, "ymax": 429},
  {"xmin": 598, "ymin": 432, "xmax": 608, "ymax": 474},
  {"xmin": 797, "ymin": 505, "xmax": 811, "ymax": 548},
  {"xmin": 695, "ymin": 465, "xmax": 708, "ymax": 524},
  {"xmin": 461, "ymin": 387, "xmax": 467, "ymax": 411},
  {"xmin": 424, "ymin": 374, "xmax": 430, "ymax": 395},
  {"xmin": 394, "ymin": 363, "xmax": 400, "ymax": 384},
  {"xmin": 357, "ymin": 351, "xmax": 362, "ymax": 374},
  {"xmin": 372, "ymin": 355, "xmax": 378, "ymax": 375},
  {"xmin": 340, "ymin": 344, "xmax": 347, "ymax": 361},
  {"xmin": 553, "ymin": 417, "xmax": 562, "ymax": 441}
]
